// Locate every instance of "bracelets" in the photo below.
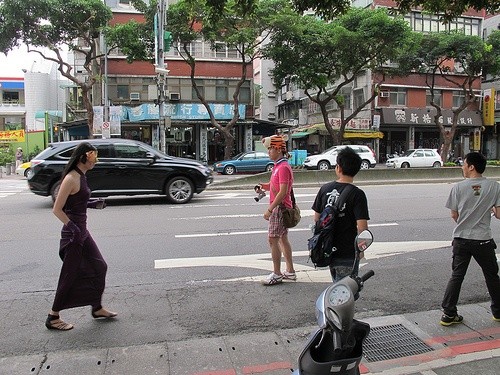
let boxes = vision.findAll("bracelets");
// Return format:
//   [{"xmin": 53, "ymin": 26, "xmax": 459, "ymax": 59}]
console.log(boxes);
[{"xmin": 268, "ymin": 209, "xmax": 273, "ymax": 213}]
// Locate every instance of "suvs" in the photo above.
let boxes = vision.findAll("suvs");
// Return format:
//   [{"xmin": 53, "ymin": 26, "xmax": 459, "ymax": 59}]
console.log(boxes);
[
  {"xmin": 385, "ymin": 148, "xmax": 444, "ymax": 169},
  {"xmin": 302, "ymin": 144, "xmax": 377, "ymax": 171},
  {"xmin": 26, "ymin": 138, "xmax": 214, "ymax": 204}
]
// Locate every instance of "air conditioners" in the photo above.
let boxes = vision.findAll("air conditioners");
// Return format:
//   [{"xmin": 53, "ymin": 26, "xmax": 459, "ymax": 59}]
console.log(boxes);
[
  {"xmin": 130, "ymin": 93, "xmax": 140, "ymax": 101},
  {"xmin": 286, "ymin": 91, "xmax": 292, "ymax": 99},
  {"xmin": 169, "ymin": 93, "xmax": 180, "ymax": 100},
  {"xmin": 380, "ymin": 91, "xmax": 389, "ymax": 97}
]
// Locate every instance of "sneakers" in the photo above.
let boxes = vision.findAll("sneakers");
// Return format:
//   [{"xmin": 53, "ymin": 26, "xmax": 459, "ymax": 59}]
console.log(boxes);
[
  {"xmin": 492, "ymin": 314, "xmax": 500, "ymax": 322},
  {"xmin": 261, "ymin": 271, "xmax": 284, "ymax": 285},
  {"xmin": 283, "ymin": 270, "xmax": 297, "ymax": 280},
  {"xmin": 440, "ymin": 311, "xmax": 463, "ymax": 327}
]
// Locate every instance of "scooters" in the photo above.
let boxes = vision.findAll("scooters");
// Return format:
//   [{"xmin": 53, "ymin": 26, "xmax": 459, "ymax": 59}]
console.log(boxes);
[{"xmin": 291, "ymin": 229, "xmax": 376, "ymax": 375}]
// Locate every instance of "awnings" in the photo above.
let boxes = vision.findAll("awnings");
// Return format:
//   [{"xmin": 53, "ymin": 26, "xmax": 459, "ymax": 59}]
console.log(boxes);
[{"xmin": 290, "ymin": 128, "xmax": 384, "ymax": 140}]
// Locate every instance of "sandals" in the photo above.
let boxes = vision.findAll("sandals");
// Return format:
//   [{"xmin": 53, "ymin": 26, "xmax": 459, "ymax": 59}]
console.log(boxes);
[
  {"xmin": 91, "ymin": 305, "xmax": 118, "ymax": 318},
  {"xmin": 44, "ymin": 313, "xmax": 74, "ymax": 331}
]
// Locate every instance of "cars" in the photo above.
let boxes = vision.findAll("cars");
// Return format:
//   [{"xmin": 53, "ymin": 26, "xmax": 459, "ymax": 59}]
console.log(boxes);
[
  {"xmin": 16, "ymin": 161, "xmax": 32, "ymax": 178},
  {"xmin": 212, "ymin": 150, "xmax": 275, "ymax": 176}
]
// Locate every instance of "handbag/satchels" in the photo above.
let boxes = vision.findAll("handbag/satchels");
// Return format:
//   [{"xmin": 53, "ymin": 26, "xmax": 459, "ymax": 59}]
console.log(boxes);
[{"xmin": 279, "ymin": 201, "xmax": 301, "ymax": 228}]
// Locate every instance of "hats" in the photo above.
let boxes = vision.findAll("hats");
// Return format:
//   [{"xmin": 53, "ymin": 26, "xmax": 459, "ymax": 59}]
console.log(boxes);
[{"xmin": 261, "ymin": 134, "xmax": 292, "ymax": 159}]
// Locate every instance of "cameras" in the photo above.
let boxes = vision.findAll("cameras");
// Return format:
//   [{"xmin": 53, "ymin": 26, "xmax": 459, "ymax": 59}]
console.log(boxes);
[{"xmin": 254, "ymin": 186, "xmax": 266, "ymax": 202}]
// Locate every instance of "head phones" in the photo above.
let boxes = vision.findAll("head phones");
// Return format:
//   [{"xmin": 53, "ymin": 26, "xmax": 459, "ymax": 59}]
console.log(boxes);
[{"xmin": 80, "ymin": 147, "xmax": 92, "ymax": 164}]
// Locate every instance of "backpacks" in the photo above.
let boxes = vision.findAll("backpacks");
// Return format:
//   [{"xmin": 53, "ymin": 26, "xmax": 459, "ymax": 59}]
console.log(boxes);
[{"xmin": 307, "ymin": 184, "xmax": 354, "ymax": 270}]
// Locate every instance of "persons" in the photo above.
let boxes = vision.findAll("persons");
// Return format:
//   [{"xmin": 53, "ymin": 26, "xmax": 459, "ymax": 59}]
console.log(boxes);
[
  {"xmin": 45, "ymin": 142, "xmax": 118, "ymax": 330},
  {"xmin": 311, "ymin": 147, "xmax": 370, "ymax": 289},
  {"xmin": 255, "ymin": 134, "xmax": 298, "ymax": 286},
  {"xmin": 439, "ymin": 153, "xmax": 500, "ymax": 327},
  {"xmin": 16, "ymin": 148, "xmax": 24, "ymax": 175}
]
